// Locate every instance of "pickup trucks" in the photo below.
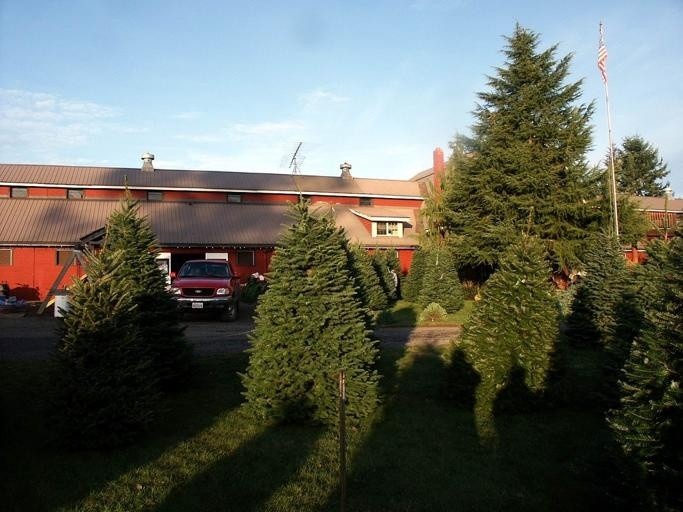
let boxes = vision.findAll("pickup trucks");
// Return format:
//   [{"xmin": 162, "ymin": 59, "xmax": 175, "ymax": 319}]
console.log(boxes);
[{"xmin": 168, "ymin": 259, "xmax": 241, "ymax": 322}]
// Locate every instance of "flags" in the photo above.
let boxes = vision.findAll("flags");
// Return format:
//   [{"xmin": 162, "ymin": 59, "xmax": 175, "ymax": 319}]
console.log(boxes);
[{"xmin": 597, "ymin": 24, "xmax": 609, "ymax": 88}]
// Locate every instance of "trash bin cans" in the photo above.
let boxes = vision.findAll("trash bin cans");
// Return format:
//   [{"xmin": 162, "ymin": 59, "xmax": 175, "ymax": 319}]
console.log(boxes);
[{"xmin": 54, "ymin": 291, "xmax": 72, "ymax": 320}]
